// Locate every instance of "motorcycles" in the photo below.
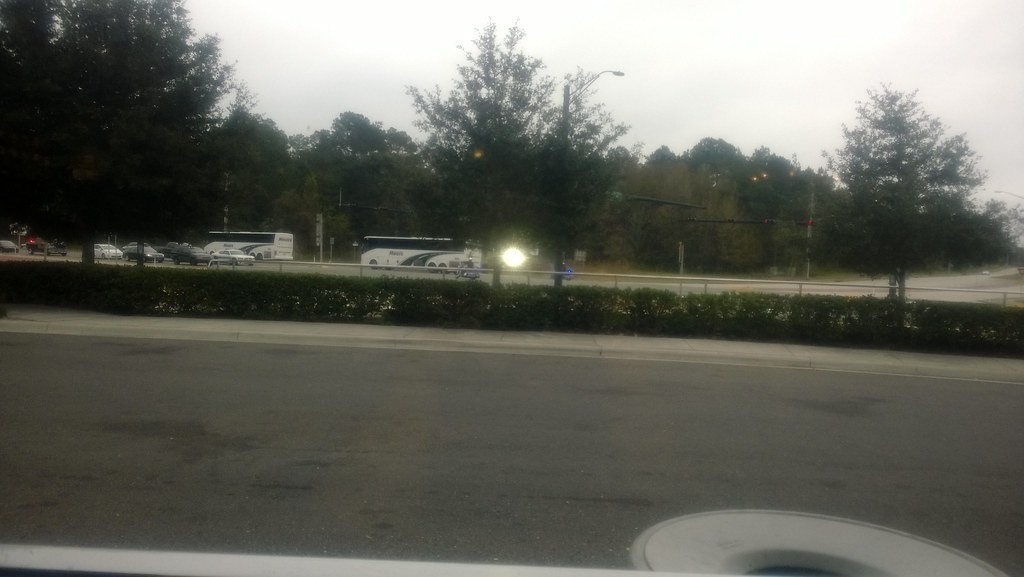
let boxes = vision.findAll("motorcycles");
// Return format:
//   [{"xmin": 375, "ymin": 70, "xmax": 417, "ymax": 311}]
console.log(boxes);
[{"xmin": 453, "ymin": 262, "xmax": 480, "ymax": 280}]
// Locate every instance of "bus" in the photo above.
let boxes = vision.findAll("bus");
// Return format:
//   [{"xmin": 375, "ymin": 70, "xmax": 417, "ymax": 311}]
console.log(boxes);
[
  {"xmin": 360, "ymin": 235, "xmax": 484, "ymax": 271},
  {"xmin": 205, "ymin": 232, "xmax": 294, "ymax": 260}
]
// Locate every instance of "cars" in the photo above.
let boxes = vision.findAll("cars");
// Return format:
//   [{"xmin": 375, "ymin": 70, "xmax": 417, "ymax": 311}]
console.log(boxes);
[
  {"xmin": 123, "ymin": 242, "xmax": 148, "ymax": 250},
  {"xmin": 123, "ymin": 246, "xmax": 165, "ymax": 263},
  {"xmin": 26, "ymin": 238, "xmax": 68, "ymax": 256},
  {"xmin": 211, "ymin": 249, "xmax": 256, "ymax": 266},
  {"xmin": 0, "ymin": 240, "xmax": 19, "ymax": 253},
  {"xmin": 94, "ymin": 244, "xmax": 123, "ymax": 259}
]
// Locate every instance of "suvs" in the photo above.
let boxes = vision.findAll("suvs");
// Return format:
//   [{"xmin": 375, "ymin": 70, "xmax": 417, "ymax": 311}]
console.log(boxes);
[
  {"xmin": 170, "ymin": 245, "xmax": 212, "ymax": 267},
  {"xmin": 165, "ymin": 242, "xmax": 189, "ymax": 258}
]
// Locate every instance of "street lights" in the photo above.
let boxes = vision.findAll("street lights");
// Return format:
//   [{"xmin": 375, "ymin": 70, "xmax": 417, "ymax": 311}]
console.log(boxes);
[{"xmin": 551, "ymin": 70, "xmax": 625, "ymax": 284}]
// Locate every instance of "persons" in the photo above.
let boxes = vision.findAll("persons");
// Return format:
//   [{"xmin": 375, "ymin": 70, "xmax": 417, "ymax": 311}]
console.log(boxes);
[{"xmin": 462, "ymin": 258, "xmax": 475, "ymax": 270}]
